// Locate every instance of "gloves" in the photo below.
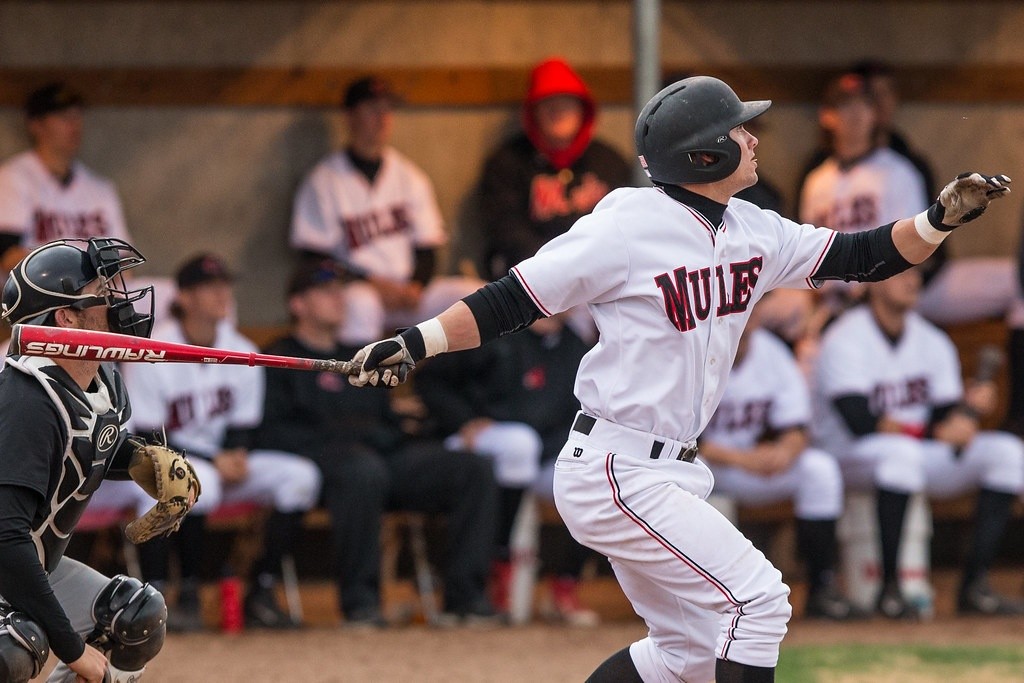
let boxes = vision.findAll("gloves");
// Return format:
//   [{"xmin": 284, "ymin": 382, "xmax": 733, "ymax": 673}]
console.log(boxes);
[
  {"xmin": 348, "ymin": 316, "xmax": 449, "ymax": 389},
  {"xmin": 913, "ymin": 171, "xmax": 1012, "ymax": 244}
]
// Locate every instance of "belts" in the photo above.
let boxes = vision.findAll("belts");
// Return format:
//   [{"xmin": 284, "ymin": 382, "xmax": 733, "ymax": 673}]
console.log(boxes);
[{"xmin": 573, "ymin": 414, "xmax": 699, "ymax": 463}]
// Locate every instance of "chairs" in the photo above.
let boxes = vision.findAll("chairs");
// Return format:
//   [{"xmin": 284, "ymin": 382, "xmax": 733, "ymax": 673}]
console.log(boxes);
[{"xmin": 72, "ymin": 321, "xmax": 1024, "ymax": 630}]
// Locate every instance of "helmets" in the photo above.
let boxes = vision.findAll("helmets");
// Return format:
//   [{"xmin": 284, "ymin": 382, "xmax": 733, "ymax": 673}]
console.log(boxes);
[
  {"xmin": 634, "ymin": 75, "xmax": 773, "ymax": 185},
  {"xmin": 0, "ymin": 240, "xmax": 98, "ymax": 357}
]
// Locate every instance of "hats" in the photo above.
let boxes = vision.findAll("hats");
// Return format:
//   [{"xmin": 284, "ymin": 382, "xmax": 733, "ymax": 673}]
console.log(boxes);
[
  {"xmin": 818, "ymin": 67, "xmax": 875, "ymax": 103},
  {"xmin": 177, "ymin": 252, "xmax": 236, "ymax": 289},
  {"xmin": 290, "ymin": 247, "xmax": 367, "ymax": 293},
  {"xmin": 26, "ymin": 82, "xmax": 82, "ymax": 120},
  {"xmin": 340, "ymin": 78, "xmax": 409, "ymax": 110}
]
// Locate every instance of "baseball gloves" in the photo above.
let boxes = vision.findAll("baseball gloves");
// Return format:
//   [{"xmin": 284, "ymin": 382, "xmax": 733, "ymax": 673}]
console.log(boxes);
[{"xmin": 124, "ymin": 445, "xmax": 202, "ymax": 546}]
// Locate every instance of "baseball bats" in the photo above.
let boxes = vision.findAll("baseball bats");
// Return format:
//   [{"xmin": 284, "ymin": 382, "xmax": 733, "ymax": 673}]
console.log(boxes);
[{"xmin": 10, "ymin": 323, "xmax": 408, "ymax": 383}]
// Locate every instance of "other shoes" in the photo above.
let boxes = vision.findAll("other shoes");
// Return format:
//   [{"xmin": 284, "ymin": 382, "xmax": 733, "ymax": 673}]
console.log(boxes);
[
  {"xmin": 243, "ymin": 593, "xmax": 298, "ymax": 635},
  {"xmin": 957, "ymin": 585, "xmax": 1019, "ymax": 617},
  {"xmin": 486, "ymin": 561, "xmax": 512, "ymax": 616},
  {"xmin": 550, "ymin": 575, "xmax": 601, "ymax": 628},
  {"xmin": 162, "ymin": 599, "xmax": 199, "ymax": 630},
  {"xmin": 876, "ymin": 588, "xmax": 920, "ymax": 621},
  {"xmin": 435, "ymin": 580, "xmax": 507, "ymax": 631},
  {"xmin": 339, "ymin": 577, "xmax": 383, "ymax": 631},
  {"xmin": 804, "ymin": 585, "xmax": 866, "ymax": 621}
]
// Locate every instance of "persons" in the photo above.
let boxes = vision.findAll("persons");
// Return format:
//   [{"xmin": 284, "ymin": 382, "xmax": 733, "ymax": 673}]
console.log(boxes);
[
  {"xmin": 348, "ymin": 77, "xmax": 1010, "ymax": 683},
  {"xmin": 1, "ymin": 84, "xmax": 324, "ymax": 633},
  {"xmin": 699, "ymin": 81, "xmax": 1023, "ymax": 617},
  {"xmin": 1, "ymin": 237, "xmax": 201, "ymax": 683},
  {"xmin": 250, "ymin": 58, "xmax": 636, "ymax": 627}
]
